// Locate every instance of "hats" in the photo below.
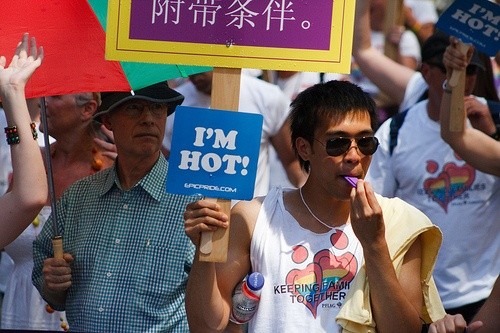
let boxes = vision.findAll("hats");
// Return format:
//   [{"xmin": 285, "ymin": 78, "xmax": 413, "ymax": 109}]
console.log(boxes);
[
  {"xmin": 91, "ymin": 81, "xmax": 185, "ymax": 123},
  {"xmin": 422, "ymin": 34, "xmax": 486, "ymax": 71}
]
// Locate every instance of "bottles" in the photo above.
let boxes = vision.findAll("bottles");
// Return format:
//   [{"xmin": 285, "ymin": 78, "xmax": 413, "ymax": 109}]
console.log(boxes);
[{"xmin": 230, "ymin": 274, "xmax": 264, "ymax": 326}]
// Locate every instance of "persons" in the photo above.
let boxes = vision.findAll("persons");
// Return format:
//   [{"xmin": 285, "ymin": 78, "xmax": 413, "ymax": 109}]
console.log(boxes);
[{"xmin": 0, "ymin": 0, "xmax": 500, "ymax": 333}]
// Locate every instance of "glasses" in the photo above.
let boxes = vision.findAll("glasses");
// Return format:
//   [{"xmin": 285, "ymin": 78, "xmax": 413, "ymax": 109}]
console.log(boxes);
[{"xmin": 313, "ymin": 137, "xmax": 379, "ymax": 157}]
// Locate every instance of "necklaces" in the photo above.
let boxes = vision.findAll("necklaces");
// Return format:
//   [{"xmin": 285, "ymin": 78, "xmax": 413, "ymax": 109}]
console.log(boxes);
[{"xmin": 299, "ymin": 187, "xmax": 334, "ymax": 229}]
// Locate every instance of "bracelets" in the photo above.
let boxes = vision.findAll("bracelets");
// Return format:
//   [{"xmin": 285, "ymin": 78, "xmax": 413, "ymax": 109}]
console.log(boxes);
[
  {"xmin": 3, "ymin": 123, "xmax": 38, "ymax": 145},
  {"xmin": 443, "ymin": 79, "xmax": 452, "ymax": 93}
]
